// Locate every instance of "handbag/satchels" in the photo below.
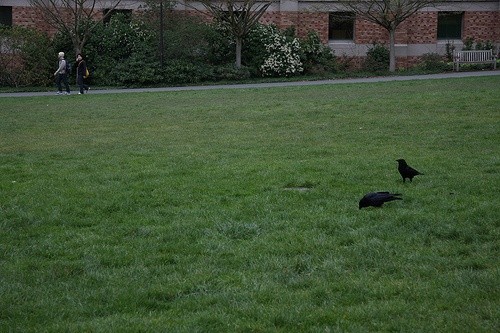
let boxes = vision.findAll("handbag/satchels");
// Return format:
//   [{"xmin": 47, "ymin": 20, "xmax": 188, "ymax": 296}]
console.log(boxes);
[{"xmin": 85, "ymin": 68, "xmax": 90, "ymax": 77}]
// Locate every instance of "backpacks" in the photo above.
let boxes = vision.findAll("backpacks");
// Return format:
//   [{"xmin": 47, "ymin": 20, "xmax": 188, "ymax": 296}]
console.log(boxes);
[{"xmin": 61, "ymin": 59, "xmax": 72, "ymax": 74}]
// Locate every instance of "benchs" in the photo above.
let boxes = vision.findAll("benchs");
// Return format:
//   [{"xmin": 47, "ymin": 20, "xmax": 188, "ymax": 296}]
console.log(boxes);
[{"xmin": 453, "ymin": 49, "xmax": 497, "ymax": 72}]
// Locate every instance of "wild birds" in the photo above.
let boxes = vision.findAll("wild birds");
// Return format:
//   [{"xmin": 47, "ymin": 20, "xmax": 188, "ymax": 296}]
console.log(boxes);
[
  {"xmin": 395, "ymin": 158, "xmax": 425, "ymax": 183},
  {"xmin": 358, "ymin": 191, "xmax": 404, "ymax": 211}
]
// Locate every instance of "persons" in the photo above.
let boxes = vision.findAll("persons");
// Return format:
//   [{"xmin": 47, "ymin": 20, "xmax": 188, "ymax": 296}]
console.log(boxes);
[
  {"xmin": 76, "ymin": 54, "xmax": 90, "ymax": 95},
  {"xmin": 54, "ymin": 52, "xmax": 70, "ymax": 95}
]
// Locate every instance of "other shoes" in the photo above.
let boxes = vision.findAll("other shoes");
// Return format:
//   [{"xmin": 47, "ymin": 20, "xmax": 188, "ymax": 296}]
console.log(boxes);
[
  {"xmin": 65, "ymin": 91, "xmax": 71, "ymax": 95},
  {"xmin": 78, "ymin": 91, "xmax": 84, "ymax": 94},
  {"xmin": 86, "ymin": 87, "xmax": 90, "ymax": 93},
  {"xmin": 56, "ymin": 92, "xmax": 63, "ymax": 95}
]
